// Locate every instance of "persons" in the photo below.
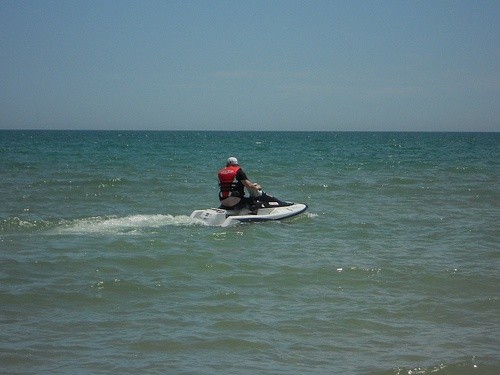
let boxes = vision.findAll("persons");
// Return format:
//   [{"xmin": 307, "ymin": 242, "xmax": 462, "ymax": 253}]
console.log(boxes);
[{"xmin": 217, "ymin": 156, "xmax": 261, "ymax": 216}]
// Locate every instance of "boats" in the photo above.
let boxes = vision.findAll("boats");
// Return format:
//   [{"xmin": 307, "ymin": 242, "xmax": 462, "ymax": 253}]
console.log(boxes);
[{"xmin": 190, "ymin": 181, "xmax": 308, "ymax": 227}]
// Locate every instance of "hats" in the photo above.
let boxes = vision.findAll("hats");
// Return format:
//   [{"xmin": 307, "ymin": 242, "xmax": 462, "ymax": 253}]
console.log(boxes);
[{"xmin": 227, "ymin": 157, "xmax": 238, "ymax": 164}]
